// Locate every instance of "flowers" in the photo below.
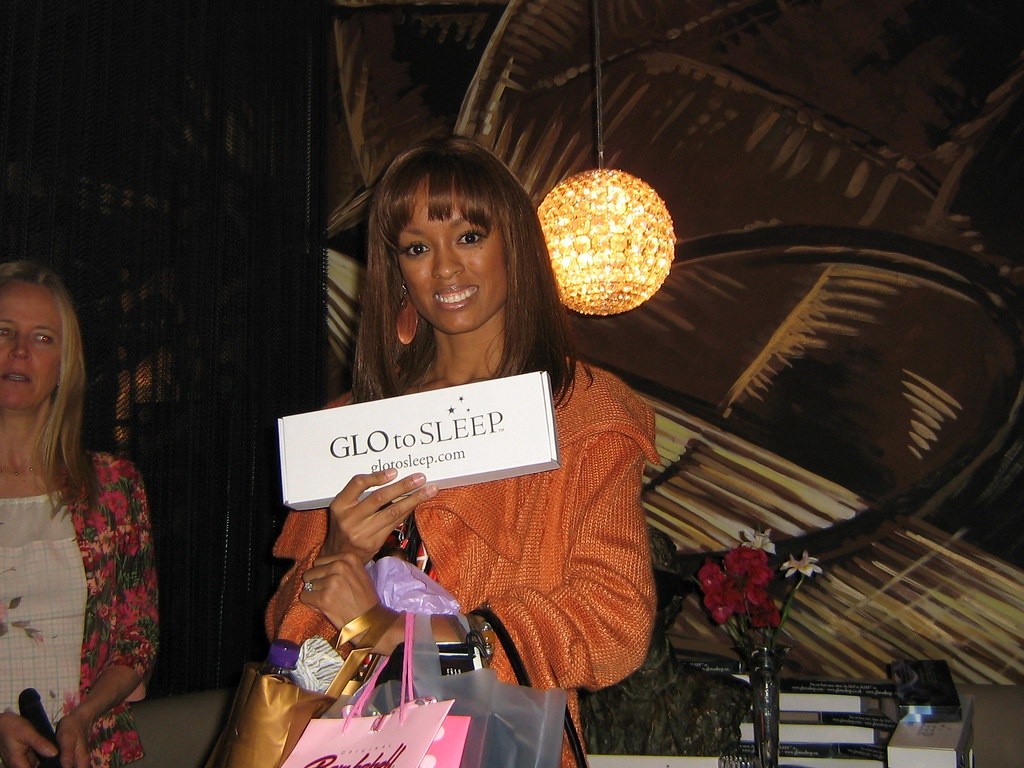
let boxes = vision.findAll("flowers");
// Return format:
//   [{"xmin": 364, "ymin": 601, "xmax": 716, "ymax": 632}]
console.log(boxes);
[{"xmin": 687, "ymin": 529, "xmax": 822, "ymax": 642}]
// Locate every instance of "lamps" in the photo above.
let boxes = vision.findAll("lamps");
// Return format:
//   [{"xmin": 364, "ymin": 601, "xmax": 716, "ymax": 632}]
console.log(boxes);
[{"xmin": 537, "ymin": 0, "xmax": 677, "ymax": 316}]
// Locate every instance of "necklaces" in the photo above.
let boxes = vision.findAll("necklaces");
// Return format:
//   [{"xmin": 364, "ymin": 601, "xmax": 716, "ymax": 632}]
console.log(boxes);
[
  {"xmin": 0, "ymin": 467, "xmax": 33, "ymax": 475},
  {"xmin": 418, "ymin": 362, "xmax": 431, "ymax": 392}
]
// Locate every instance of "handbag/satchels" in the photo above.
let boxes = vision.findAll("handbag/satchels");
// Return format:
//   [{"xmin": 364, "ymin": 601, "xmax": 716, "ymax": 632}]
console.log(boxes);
[{"xmin": 200, "ymin": 603, "xmax": 565, "ymax": 768}]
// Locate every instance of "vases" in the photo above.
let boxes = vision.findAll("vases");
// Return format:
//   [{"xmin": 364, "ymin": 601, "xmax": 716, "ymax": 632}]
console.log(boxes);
[{"xmin": 730, "ymin": 645, "xmax": 791, "ymax": 768}]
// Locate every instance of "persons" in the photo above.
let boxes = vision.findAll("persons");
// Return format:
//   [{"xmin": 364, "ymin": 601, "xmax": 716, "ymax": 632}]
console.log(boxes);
[
  {"xmin": 0, "ymin": 258, "xmax": 158, "ymax": 768},
  {"xmin": 263, "ymin": 136, "xmax": 661, "ymax": 768}
]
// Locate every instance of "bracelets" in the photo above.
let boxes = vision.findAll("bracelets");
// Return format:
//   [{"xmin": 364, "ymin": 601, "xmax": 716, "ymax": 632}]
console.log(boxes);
[{"xmin": 481, "ymin": 622, "xmax": 492, "ymax": 659}]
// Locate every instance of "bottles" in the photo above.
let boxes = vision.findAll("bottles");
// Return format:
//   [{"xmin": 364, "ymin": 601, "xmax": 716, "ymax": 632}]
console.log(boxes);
[{"xmin": 259, "ymin": 638, "xmax": 305, "ymax": 688}]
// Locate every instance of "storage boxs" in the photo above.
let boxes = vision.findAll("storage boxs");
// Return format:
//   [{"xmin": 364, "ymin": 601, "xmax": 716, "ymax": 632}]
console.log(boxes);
[
  {"xmin": 779, "ymin": 692, "xmax": 879, "ymax": 714},
  {"xmin": 887, "ymin": 695, "xmax": 974, "ymax": 768},
  {"xmin": 277, "ymin": 370, "xmax": 561, "ymax": 511},
  {"xmin": 739, "ymin": 723, "xmax": 880, "ymax": 744},
  {"xmin": 779, "ymin": 711, "xmax": 822, "ymax": 725}
]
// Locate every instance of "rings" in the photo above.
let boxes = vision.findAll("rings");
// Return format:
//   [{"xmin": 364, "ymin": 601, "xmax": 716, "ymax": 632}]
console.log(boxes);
[{"xmin": 303, "ymin": 581, "xmax": 312, "ymax": 590}]
